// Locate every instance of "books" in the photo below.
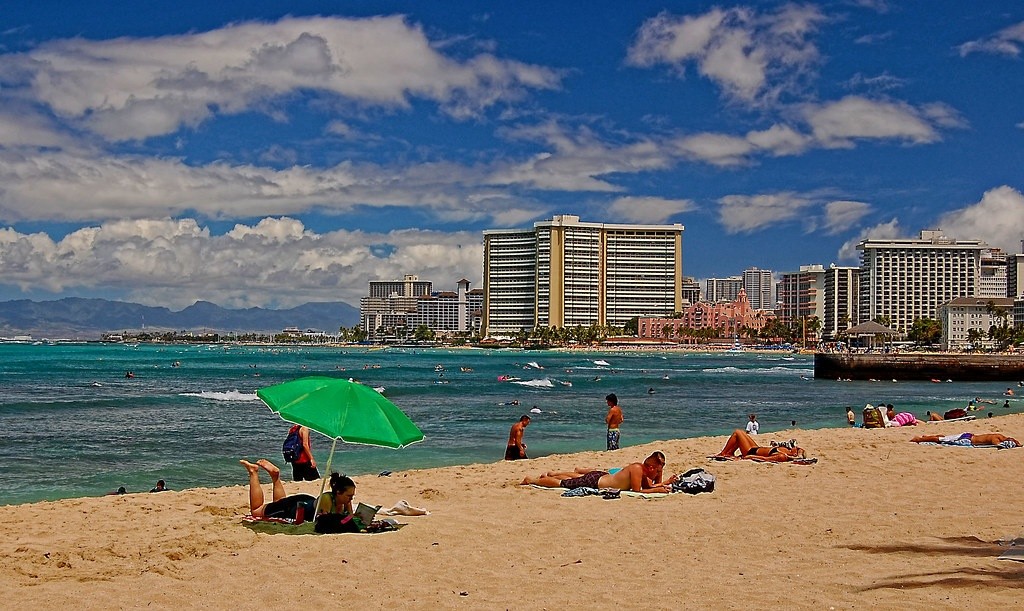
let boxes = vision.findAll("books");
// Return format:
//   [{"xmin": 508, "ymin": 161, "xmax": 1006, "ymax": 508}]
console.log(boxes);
[{"xmin": 355, "ymin": 502, "xmax": 382, "ymax": 525}]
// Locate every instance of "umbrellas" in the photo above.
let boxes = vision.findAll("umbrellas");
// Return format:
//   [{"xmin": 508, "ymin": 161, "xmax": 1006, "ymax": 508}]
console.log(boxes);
[{"xmin": 257, "ymin": 375, "xmax": 426, "ymax": 524}]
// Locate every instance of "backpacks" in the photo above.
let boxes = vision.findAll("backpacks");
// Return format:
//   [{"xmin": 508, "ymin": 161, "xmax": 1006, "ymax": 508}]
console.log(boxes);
[{"xmin": 282, "ymin": 425, "xmax": 301, "ymax": 461}]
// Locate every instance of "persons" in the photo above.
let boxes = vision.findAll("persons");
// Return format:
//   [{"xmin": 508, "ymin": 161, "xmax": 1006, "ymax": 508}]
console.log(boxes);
[
  {"xmin": 288, "ymin": 425, "xmax": 321, "ymax": 482},
  {"xmin": 239, "ymin": 459, "xmax": 375, "ymax": 521},
  {"xmin": 910, "ymin": 432, "xmax": 1021, "ymax": 447},
  {"xmin": 863, "ymin": 403, "xmax": 918, "ymax": 425},
  {"xmin": 150, "ymin": 479, "xmax": 168, "ymax": 492},
  {"xmin": 605, "ymin": 393, "xmax": 623, "ymax": 450},
  {"xmin": 521, "ymin": 450, "xmax": 678, "ymax": 495},
  {"xmin": 115, "ymin": 487, "xmax": 127, "ymax": 494},
  {"xmin": 745, "ymin": 414, "xmax": 760, "ymax": 434},
  {"xmin": 927, "ymin": 411, "xmax": 943, "ymax": 421},
  {"xmin": 125, "ymin": 371, "xmax": 133, "ymax": 377},
  {"xmin": 716, "ymin": 430, "xmax": 807, "ymax": 461},
  {"xmin": 505, "ymin": 415, "xmax": 530, "ymax": 460},
  {"xmin": 1006, "ymin": 388, "xmax": 1014, "ymax": 395},
  {"xmin": 846, "ymin": 407, "xmax": 855, "ymax": 424},
  {"xmin": 968, "ymin": 402, "xmax": 977, "ymax": 410}
]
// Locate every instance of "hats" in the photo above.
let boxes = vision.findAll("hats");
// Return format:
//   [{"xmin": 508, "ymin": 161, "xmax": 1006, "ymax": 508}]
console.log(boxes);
[{"xmin": 606, "ymin": 394, "xmax": 617, "ymax": 405}]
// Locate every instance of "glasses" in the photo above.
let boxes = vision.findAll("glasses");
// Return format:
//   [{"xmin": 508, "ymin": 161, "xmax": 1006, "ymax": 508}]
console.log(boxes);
[{"xmin": 797, "ymin": 448, "xmax": 800, "ymax": 455}]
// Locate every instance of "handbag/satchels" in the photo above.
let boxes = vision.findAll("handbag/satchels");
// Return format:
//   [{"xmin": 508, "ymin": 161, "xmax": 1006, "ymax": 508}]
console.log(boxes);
[{"xmin": 315, "ymin": 513, "xmax": 354, "ymax": 533}]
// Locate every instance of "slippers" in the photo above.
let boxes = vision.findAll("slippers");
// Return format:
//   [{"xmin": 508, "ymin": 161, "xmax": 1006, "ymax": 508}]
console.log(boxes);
[
  {"xmin": 380, "ymin": 520, "xmax": 397, "ymax": 531},
  {"xmin": 707, "ymin": 456, "xmax": 732, "ymax": 461},
  {"xmin": 603, "ymin": 492, "xmax": 621, "ymax": 499},
  {"xmin": 367, "ymin": 521, "xmax": 380, "ymax": 532}
]
